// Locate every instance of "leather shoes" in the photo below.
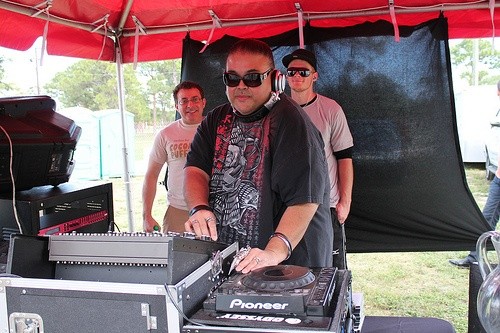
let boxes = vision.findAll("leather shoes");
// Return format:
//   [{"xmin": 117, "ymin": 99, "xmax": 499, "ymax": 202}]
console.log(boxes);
[{"xmin": 449, "ymin": 255, "xmax": 479, "ymax": 268}]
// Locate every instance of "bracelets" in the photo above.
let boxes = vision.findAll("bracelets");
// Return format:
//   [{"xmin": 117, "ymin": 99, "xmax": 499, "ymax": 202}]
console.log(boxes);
[
  {"xmin": 188, "ymin": 205, "xmax": 215, "ymax": 217},
  {"xmin": 269, "ymin": 232, "xmax": 292, "ymax": 261}
]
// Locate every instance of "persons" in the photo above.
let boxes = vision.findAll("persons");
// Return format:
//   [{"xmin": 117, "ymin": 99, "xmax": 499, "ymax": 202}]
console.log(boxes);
[
  {"xmin": 183, "ymin": 39, "xmax": 333, "ymax": 274},
  {"xmin": 143, "ymin": 81, "xmax": 206, "ymax": 235},
  {"xmin": 449, "ymin": 80, "xmax": 500, "ymax": 269},
  {"xmin": 282, "ymin": 48, "xmax": 353, "ymax": 270}
]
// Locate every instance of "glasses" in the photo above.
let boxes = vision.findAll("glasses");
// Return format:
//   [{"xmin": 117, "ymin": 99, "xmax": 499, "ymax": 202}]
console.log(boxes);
[
  {"xmin": 286, "ymin": 66, "xmax": 315, "ymax": 78},
  {"xmin": 177, "ymin": 97, "xmax": 203, "ymax": 106},
  {"xmin": 222, "ymin": 67, "xmax": 272, "ymax": 87}
]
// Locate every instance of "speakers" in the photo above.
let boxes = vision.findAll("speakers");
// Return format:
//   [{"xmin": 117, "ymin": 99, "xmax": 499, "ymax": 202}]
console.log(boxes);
[{"xmin": 0, "ymin": 96, "xmax": 82, "ymax": 195}]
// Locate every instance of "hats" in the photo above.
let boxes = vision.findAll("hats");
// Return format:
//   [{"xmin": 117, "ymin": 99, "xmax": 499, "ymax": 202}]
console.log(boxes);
[{"xmin": 281, "ymin": 48, "xmax": 317, "ymax": 72}]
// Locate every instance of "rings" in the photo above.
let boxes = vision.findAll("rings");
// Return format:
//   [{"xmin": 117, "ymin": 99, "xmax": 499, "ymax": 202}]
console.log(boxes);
[
  {"xmin": 254, "ymin": 256, "xmax": 261, "ymax": 263},
  {"xmin": 206, "ymin": 217, "xmax": 214, "ymax": 222},
  {"xmin": 191, "ymin": 219, "xmax": 199, "ymax": 225}
]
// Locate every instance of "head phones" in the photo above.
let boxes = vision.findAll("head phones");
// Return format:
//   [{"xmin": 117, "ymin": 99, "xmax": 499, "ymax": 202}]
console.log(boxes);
[{"xmin": 232, "ymin": 69, "xmax": 286, "ymax": 123}]
